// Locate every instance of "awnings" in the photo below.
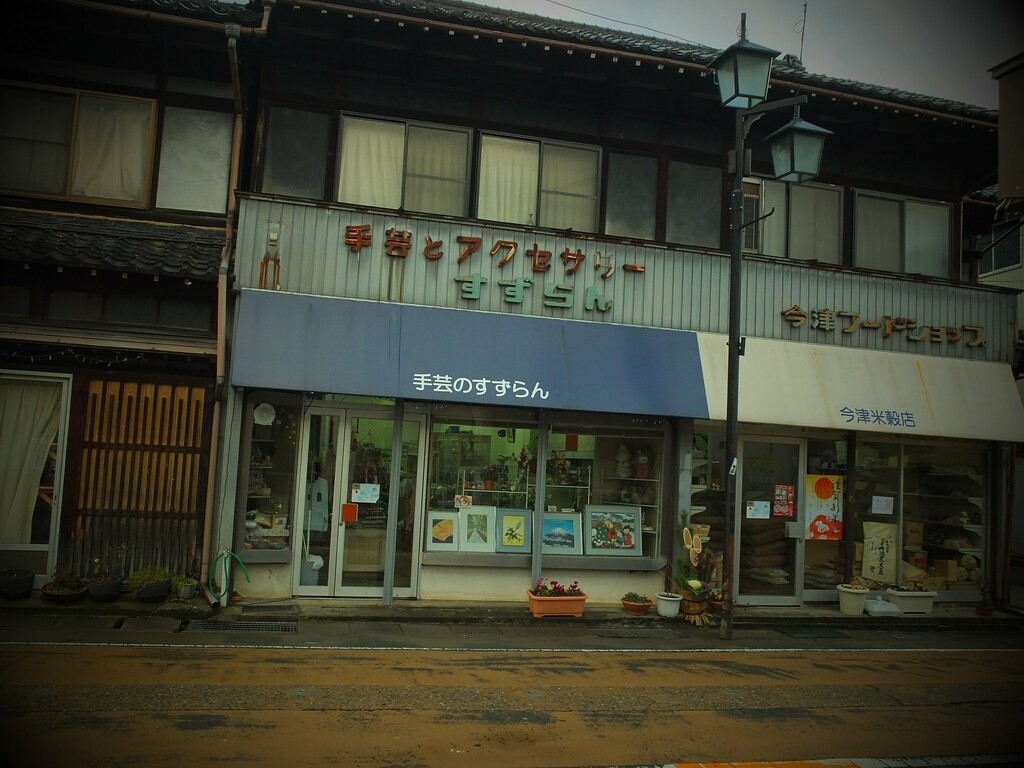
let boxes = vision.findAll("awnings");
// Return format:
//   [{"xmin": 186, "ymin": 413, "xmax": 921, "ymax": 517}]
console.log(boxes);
[
  {"xmin": 230, "ymin": 286, "xmax": 1024, "ymax": 461},
  {"xmin": 2, "ymin": 208, "xmax": 237, "ymax": 285},
  {"xmin": 143, "ymin": 0, "xmax": 1003, "ymax": 133}
]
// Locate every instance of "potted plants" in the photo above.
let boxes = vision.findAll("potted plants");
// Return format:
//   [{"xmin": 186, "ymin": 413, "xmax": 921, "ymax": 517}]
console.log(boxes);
[
  {"xmin": 41, "ymin": 566, "xmax": 88, "ymax": 602},
  {"xmin": 171, "ymin": 575, "xmax": 201, "ymax": 599},
  {"xmin": 655, "ymin": 576, "xmax": 683, "ymax": 616},
  {"xmin": 125, "ymin": 562, "xmax": 171, "ymax": 604},
  {"xmin": 477, "ymin": 469, "xmax": 498, "ymax": 490},
  {"xmin": 974, "ymin": 588, "xmax": 996, "ymax": 617},
  {"xmin": 86, "ymin": 541, "xmax": 129, "ymax": 602},
  {"xmin": 621, "ymin": 592, "xmax": 654, "ymax": 615}
]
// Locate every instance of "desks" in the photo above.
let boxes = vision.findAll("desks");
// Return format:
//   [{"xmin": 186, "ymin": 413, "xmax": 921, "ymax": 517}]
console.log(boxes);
[{"xmin": 343, "ymin": 528, "xmax": 387, "ymax": 582}]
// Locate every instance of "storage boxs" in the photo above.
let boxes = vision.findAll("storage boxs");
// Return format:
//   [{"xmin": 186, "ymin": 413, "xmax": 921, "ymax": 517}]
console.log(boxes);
[
  {"xmin": 903, "ymin": 521, "xmax": 923, "ymax": 547},
  {"xmin": 255, "ymin": 425, "xmax": 273, "ymax": 439},
  {"xmin": 837, "ymin": 540, "xmax": 864, "ymax": 560},
  {"xmin": 934, "ymin": 559, "xmax": 957, "ymax": 581},
  {"xmin": 902, "ymin": 551, "xmax": 928, "ymax": 570},
  {"xmin": 273, "ymin": 513, "xmax": 288, "ymax": 528},
  {"xmin": 693, "ymin": 450, "xmax": 705, "ymax": 459}
]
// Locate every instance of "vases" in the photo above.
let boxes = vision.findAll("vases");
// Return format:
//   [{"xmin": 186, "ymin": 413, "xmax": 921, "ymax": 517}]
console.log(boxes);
[
  {"xmin": 477, "ymin": 481, "xmax": 485, "ymax": 489},
  {"xmin": 0, "ymin": 569, "xmax": 37, "ymax": 600},
  {"xmin": 837, "ymin": 584, "xmax": 870, "ymax": 616},
  {"xmin": 706, "ymin": 599, "xmax": 737, "ymax": 614},
  {"xmin": 528, "ymin": 472, "xmax": 536, "ymax": 484},
  {"xmin": 886, "ymin": 588, "xmax": 938, "ymax": 613},
  {"xmin": 527, "ymin": 589, "xmax": 588, "ymax": 617},
  {"xmin": 675, "ymin": 586, "xmax": 710, "ymax": 616},
  {"xmin": 545, "ymin": 473, "xmax": 561, "ymax": 485}
]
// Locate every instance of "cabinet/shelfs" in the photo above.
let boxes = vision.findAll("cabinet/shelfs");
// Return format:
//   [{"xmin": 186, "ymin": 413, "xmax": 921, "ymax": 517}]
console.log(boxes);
[
  {"xmin": 691, "ymin": 458, "xmax": 708, "ymax": 514},
  {"xmin": 497, "ymin": 463, "xmax": 519, "ymax": 481},
  {"xmin": 598, "ymin": 463, "xmax": 661, "ymax": 557},
  {"xmin": 436, "ymin": 433, "xmax": 492, "ymax": 487},
  {"xmin": 457, "ymin": 462, "xmax": 527, "ymax": 509},
  {"xmin": 856, "ymin": 466, "xmax": 987, "ymax": 588},
  {"xmin": 251, "ymin": 438, "xmax": 275, "ymax": 499},
  {"xmin": 516, "ymin": 464, "xmax": 592, "ymax": 510}
]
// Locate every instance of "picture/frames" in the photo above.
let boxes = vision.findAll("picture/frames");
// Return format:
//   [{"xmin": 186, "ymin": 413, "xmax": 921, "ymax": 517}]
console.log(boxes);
[
  {"xmin": 425, "ymin": 509, "xmax": 458, "ymax": 552},
  {"xmin": 583, "ymin": 503, "xmax": 643, "ymax": 557},
  {"xmin": 495, "ymin": 508, "xmax": 532, "ymax": 553},
  {"xmin": 458, "ymin": 505, "xmax": 496, "ymax": 552},
  {"xmin": 532, "ymin": 511, "xmax": 583, "ymax": 556}
]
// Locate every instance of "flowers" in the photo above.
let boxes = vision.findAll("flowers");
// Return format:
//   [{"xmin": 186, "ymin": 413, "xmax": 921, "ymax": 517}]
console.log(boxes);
[
  {"xmin": 530, "ymin": 577, "xmax": 583, "ymax": 596},
  {"xmin": 709, "ymin": 590, "xmax": 736, "ymax": 603},
  {"xmin": 657, "ymin": 509, "xmax": 717, "ymax": 596},
  {"xmin": 517, "ymin": 444, "xmax": 573, "ymax": 483},
  {"xmin": 842, "ymin": 580, "xmax": 865, "ymax": 590},
  {"xmin": 890, "ymin": 582, "xmax": 931, "ymax": 592}
]
